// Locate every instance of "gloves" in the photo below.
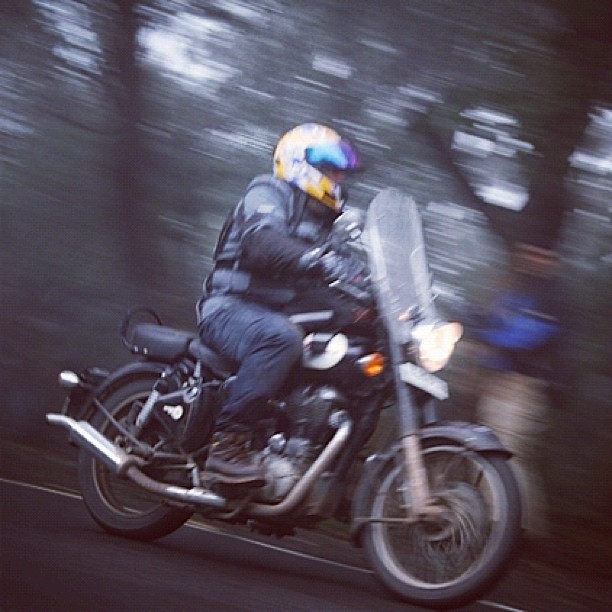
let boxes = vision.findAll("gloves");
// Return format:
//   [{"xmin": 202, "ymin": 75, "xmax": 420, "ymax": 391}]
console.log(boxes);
[{"xmin": 316, "ymin": 250, "xmax": 372, "ymax": 294}]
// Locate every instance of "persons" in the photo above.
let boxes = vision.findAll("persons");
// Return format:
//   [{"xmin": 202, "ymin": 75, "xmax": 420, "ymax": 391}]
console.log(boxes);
[
  {"xmin": 196, "ymin": 123, "xmax": 379, "ymax": 489},
  {"xmin": 455, "ymin": 206, "xmax": 582, "ymax": 551}
]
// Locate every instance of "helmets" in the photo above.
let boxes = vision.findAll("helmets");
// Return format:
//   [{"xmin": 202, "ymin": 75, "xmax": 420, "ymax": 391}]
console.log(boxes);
[{"xmin": 274, "ymin": 123, "xmax": 361, "ymax": 214}]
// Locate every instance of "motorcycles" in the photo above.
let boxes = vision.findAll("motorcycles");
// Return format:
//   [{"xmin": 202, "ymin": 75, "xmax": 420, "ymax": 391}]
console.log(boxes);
[{"xmin": 45, "ymin": 183, "xmax": 521, "ymax": 610}]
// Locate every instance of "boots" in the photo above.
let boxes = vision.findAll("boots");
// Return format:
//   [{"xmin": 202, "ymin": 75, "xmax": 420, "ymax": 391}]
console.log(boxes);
[{"xmin": 201, "ymin": 424, "xmax": 267, "ymax": 486}]
